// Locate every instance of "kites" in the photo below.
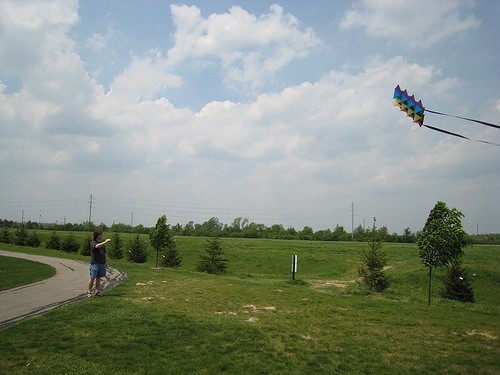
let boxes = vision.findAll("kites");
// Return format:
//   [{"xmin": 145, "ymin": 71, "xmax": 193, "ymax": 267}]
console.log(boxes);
[{"xmin": 391, "ymin": 84, "xmax": 498, "ymax": 146}]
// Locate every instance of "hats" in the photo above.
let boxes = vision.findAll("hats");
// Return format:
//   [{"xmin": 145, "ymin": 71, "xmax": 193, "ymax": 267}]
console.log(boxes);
[{"xmin": 93, "ymin": 231, "xmax": 102, "ymax": 237}]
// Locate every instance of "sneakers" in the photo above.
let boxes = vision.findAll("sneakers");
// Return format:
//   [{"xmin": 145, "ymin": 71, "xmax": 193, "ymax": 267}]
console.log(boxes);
[
  {"xmin": 94, "ymin": 290, "xmax": 103, "ymax": 296},
  {"xmin": 87, "ymin": 292, "xmax": 92, "ymax": 298}
]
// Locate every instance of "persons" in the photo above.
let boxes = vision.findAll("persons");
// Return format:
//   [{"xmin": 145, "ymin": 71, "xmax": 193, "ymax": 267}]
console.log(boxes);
[{"xmin": 87, "ymin": 231, "xmax": 110, "ymax": 298}]
